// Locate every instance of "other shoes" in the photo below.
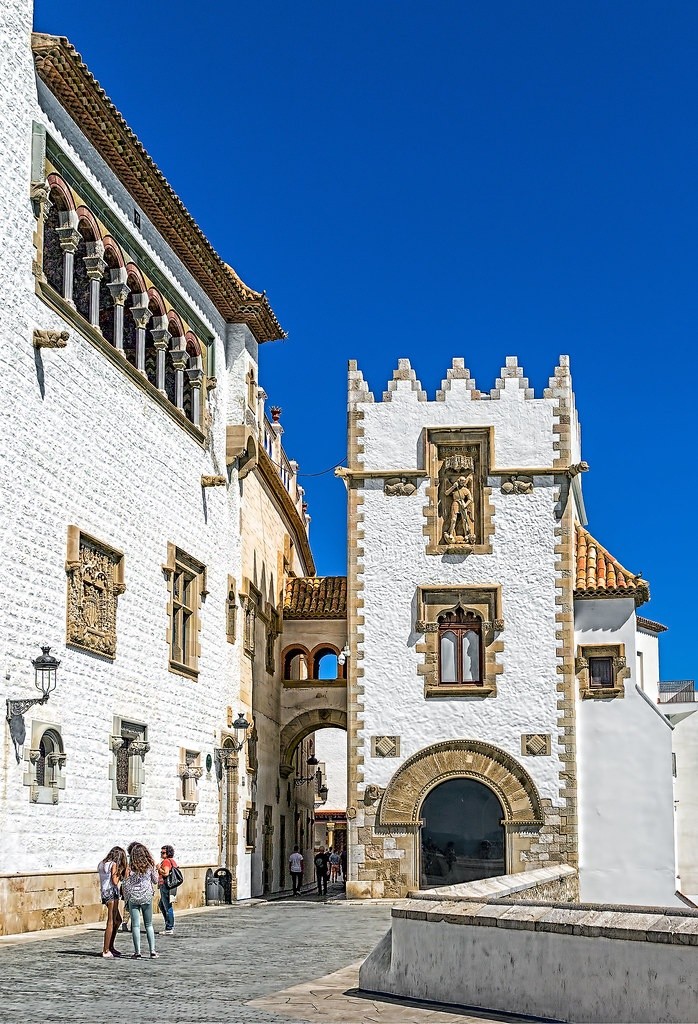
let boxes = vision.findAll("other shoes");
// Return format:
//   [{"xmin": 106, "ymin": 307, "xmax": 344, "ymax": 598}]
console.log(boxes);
[
  {"xmin": 103, "ymin": 951, "xmax": 115, "ymax": 960},
  {"xmin": 159, "ymin": 930, "xmax": 173, "ymax": 935},
  {"xmin": 122, "ymin": 923, "xmax": 129, "ymax": 931},
  {"xmin": 150, "ymin": 951, "xmax": 159, "ymax": 959},
  {"xmin": 131, "ymin": 953, "xmax": 141, "ymax": 960},
  {"xmin": 294, "ymin": 895, "xmax": 297, "ymax": 898},
  {"xmin": 110, "ymin": 948, "xmax": 121, "ymax": 957},
  {"xmin": 323, "ymin": 891, "xmax": 327, "ymax": 895},
  {"xmin": 317, "ymin": 891, "xmax": 322, "ymax": 896},
  {"xmin": 296, "ymin": 891, "xmax": 301, "ymax": 897}
]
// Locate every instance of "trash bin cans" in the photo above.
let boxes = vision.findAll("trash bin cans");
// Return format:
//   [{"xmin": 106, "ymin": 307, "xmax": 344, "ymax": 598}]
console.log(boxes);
[{"xmin": 206, "ymin": 877, "xmax": 221, "ymax": 901}]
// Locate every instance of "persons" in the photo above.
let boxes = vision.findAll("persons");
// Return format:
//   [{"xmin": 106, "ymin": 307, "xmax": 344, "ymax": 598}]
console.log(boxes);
[
  {"xmin": 288, "ymin": 846, "xmax": 304, "ymax": 897},
  {"xmin": 443, "ymin": 841, "xmax": 456, "ymax": 872},
  {"xmin": 445, "ymin": 476, "xmax": 472, "ymax": 542},
  {"xmin": 314, "ymin": 846, "xmax": 330, "ymax": 896},
  {"xmin": 97, "ymin": 841, "xmax": 178, "ymax": 959},
  {"xmin": 325, "ymin": 847, "xmax": 347, "ymax": 883}
]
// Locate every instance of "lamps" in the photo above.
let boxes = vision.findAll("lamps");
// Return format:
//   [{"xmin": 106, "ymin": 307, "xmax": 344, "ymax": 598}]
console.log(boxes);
[
  {"xmin": 293, "ymin": 754, "xmax": 320, "ymax": 789},
  {"xmin": 214, "ymin": 713, "xmax": 250, "ymax": 779},
  {"xmin": 6, "ymin": 646, "xmax": 61, "ymax": 722},
  {"xmin": 313, "ymin": 785, "xmax": 329, "ymax": 809}
]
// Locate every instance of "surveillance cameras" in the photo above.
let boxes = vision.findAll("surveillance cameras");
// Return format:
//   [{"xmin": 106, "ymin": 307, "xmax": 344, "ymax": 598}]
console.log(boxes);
[{"xmin": 337, "ymin": 654, "xmax": 345, "ymax": 665}]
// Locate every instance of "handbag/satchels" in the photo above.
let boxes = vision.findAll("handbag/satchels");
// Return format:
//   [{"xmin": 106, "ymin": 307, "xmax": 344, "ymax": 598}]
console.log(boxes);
[{"xmin": 162, "ymin": 859, "xmax": 183, "ymax": 890}]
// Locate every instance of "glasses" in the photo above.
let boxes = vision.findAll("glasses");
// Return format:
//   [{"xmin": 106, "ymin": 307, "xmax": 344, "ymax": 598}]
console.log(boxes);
[{"xmin": 161, "ymin": 851, "xmax": 166, "ymax": 855}]
